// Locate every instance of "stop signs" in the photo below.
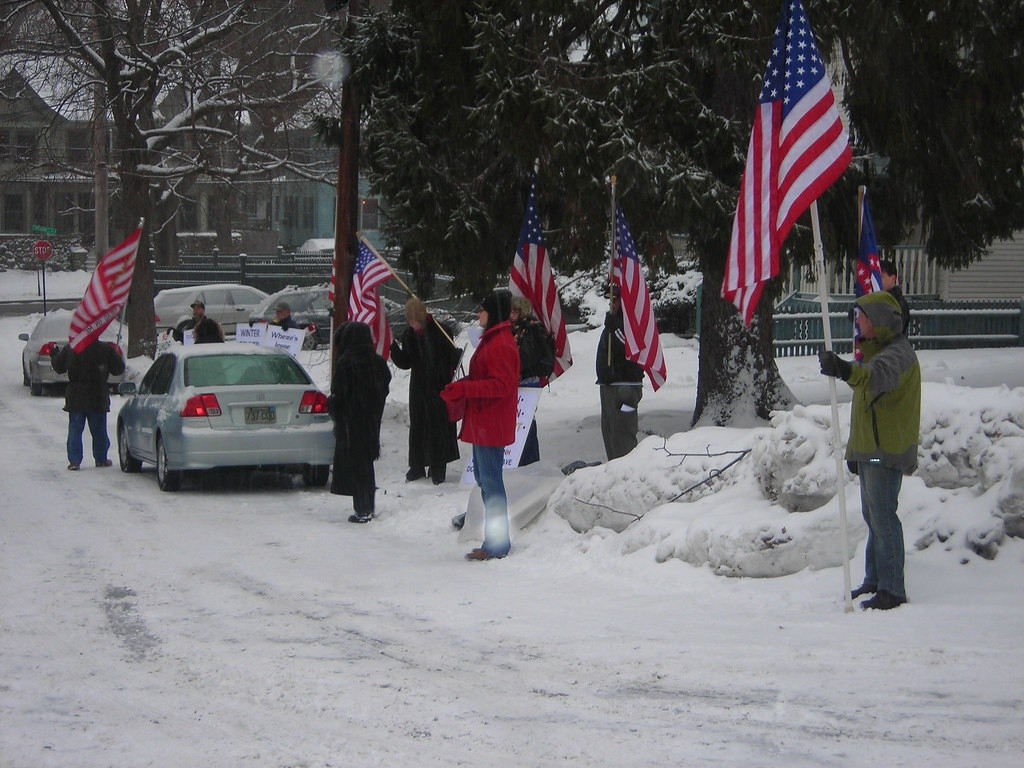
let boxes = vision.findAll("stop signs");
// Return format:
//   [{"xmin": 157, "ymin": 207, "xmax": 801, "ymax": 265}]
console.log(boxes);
[{"xmin": 33, "ymin": 240, "xmax": 52, "ymax": 262}]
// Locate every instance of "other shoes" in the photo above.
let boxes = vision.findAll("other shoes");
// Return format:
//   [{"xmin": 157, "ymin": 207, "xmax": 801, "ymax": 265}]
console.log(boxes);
[
  {"xmin": 94, "ymin": 460, "xmax": 113, "ymax": 467},
  {"xmin": 67, "ymin": 463, "xmax": 81, "ymax": 470},
  {"xmin": 851, "ymin": 583, "xmax": 876, "ymax": 600},
  {"xmin": 465, "ymin": 548, "xmax": 503, "ymax": 561},
  {"xmin": 348, "ymin": 511, "xmax": 374, "ymax": 523},
  {"xmin": 860, "ymin": 589, "xmax": 905, "ymax": 611},
  {"xmin": 406, "ymin": 466, "xmax": 426, "ymax": 481},
  {"xmin": 433, "ymin": 473, "xmax": 446, "ymax": 485}
]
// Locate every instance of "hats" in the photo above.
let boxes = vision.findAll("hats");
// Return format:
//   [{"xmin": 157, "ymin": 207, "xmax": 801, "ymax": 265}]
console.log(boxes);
[
  {"xmin": 404, "ymin": 299, "xmax": 427, "ymax": 321},
  {"xmin": 190, "ymin": 301, "xmax": 205, "ymax": 310},
  {"xmin": 604, "ymin": 286, "xmax": 622, "ymax": 300},
  {"xmin": 482, "ymin": 289, "xmax": 513, "ymax": 322},
  {"xmin": 511, "ymin": 295, "xmax": 532, "ymax": 318},
  {"xmin": 272, "ymin": 301, "xmax": 290, "ymax": 311}
]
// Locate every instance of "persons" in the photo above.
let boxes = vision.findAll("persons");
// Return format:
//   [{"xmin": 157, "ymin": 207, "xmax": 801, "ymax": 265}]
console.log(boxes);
[
  {"xmin": 181, "ymin": 301, "xmax": 224, "ymax": 344},
  {"xmin": 50, "ymin": 337, "xmax": 124, "ymax": 469},
  {"xmin": 818, "ymin": 292, "xmax": 922, "ymax": 610},
  {"xmin": 509, "ymin": 297, "xmax": 556, "ymax": 466},
  {"xmin": 391, "ymin": 298, "xmax": 461, "ymax": 484},
  {"xmin": 327, "ymin": 321, "xmax": 390, "ymax": 524},
  {"xmin": 881, "ymin": 261, "xmax": 909, "ymax": 337},
  {"xmin": 595, "ymin": 281, "xmax": 644, "ymax": 462},
  {"xmin": 439, "ymin": 288, "xmax": 521, "ymax": 562},
  {"xmin": 271, "ymin": 301, "xmax": 299, "ymax": 331}
]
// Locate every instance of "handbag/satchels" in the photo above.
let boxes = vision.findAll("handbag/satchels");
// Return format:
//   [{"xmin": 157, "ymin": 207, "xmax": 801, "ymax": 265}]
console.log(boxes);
[{"xmin": 441, "ymin": 363, "xmax": 471, "ymax": 423}]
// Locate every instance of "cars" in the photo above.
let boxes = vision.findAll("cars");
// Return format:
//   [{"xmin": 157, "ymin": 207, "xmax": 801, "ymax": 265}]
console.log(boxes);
[
  {"xmin": 248, "ymin": 284, "xmax": 463, "ymax": 351},
  {"xmin": 17, "ymin": 311, "xmax": 126, "ymax": 396},
  {"xmin": 153, "ymin": 283, "xmax": 273, "ymax": 341},
  {"xmin": 297, "ymin": 238, "xmax": 335, "ymax": 253},
  {"xmin": 115, "ymin": 343, "xmax": 337, "ymax": 492}
]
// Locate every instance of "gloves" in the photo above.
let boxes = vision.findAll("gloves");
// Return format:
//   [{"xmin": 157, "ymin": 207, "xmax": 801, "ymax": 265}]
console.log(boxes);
[
  {"xmin": 846, "ymin": 459, "xmax": 859, "ymax": 475},
  {"xmin": 604, "ymin": 312, "xmax": 620, "ymax": 332},
  {"xmin": 445, "ymin": 383, "xmax": 464, "ymax": 402},
  {"xmin": 49, "ymin": 345, "xmax": 60, "ymax": 356},
  {"xmin": 389, "ymin": 340, "xmax": 400, "ymax": 355},
  {"xmin": 817, "ymin": 351, "xmax": 851, "ymax": 382}
]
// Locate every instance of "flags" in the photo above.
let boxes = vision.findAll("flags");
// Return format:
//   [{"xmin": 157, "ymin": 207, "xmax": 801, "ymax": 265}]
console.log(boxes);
[
  {"xmin": 509, "ymin": 169, "xmax": 574, "ymax": 388},
  {"xmin": 854, "ymin": 190, "xmax": 881, "ymax": 371},
  {"xmin": 328, "ymin": 237, "xmax": 394, "ymax": 360},
  {"xmin": 612, "ymin": 188, "xmax": 666, "ymax": 393},
  {"xmin": 67, "ymin": 228, "xmax": 144, "ymax": 354},
  {"xmin": 721, "ymin": 0, "xmax": 851, "ymax": 330}
]
